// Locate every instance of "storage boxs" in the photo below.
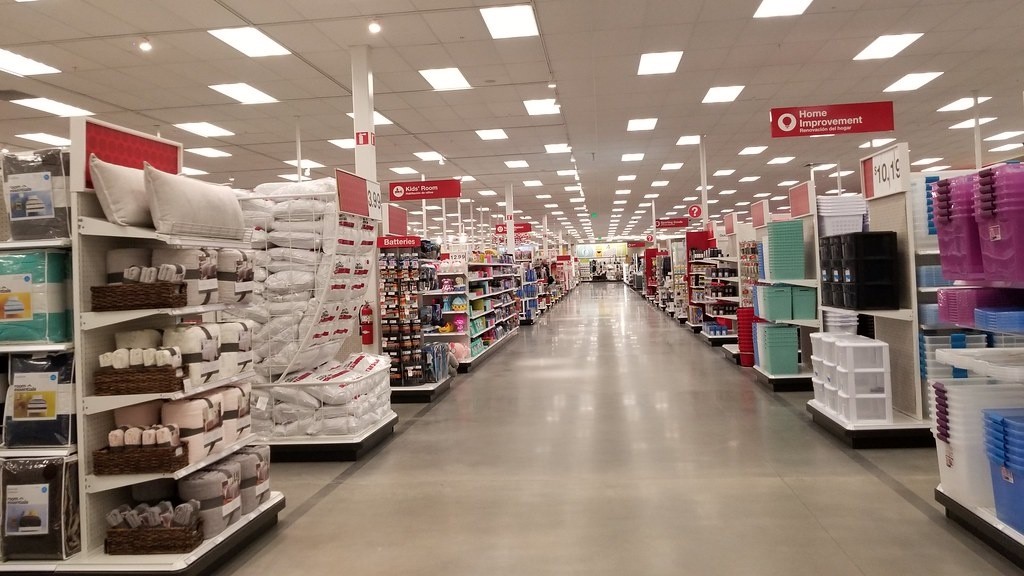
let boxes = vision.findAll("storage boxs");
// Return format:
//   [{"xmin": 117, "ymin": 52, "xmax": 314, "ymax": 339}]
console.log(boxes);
[
  {"xmin": 92, "ymin": 440, "xmax": 190, "ymax": 477},
  {"xmin": 88, "ymin": 281, "xmax": 188, "ymax": 309},
  {"xmin": 736, "ymin": 195, "xmax": 900, "ymax": 421},
  {"xmin": 911, "ymin": 170, "xmax": 1024, "ymax": 534},
  {"xmin": 738, "ymin": 196, "xmax": 867, "ymax": 375},
  {"xmin": 104, "ymin": 515, "xmax": 204, "ymax": 555},
  {"xmin": 90, "ymin": 366, "xmax": 183, "ymax": 397},
  {"xmin": 77, "ymin": 215, "xmax": 280, "ymax": 566}
]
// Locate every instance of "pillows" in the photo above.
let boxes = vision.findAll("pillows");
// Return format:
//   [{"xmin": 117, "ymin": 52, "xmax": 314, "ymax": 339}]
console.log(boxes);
[
  {"xmin": 89, "ymin": 152, "xmax": 151, "ymax": 229},
  {"xmin": 143, "ymin": 161, "xmax": 246, "ymax": 240}
]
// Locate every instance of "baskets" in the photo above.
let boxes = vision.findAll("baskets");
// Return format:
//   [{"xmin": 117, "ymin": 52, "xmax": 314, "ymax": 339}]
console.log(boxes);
[
  {"xmin": 90, "ymin": 281, "xmax": 187, "ymax": 312},
  {"xmin": 104, "ymin": 517, "xmax": 204, "ymax": 555},
  {"xmin": 91, "ymin": 364, "xmax": 184, "ymax": 396},
  {"xmin": 92, "ymin": 440, "xmax": 190, "ymax": 476}
]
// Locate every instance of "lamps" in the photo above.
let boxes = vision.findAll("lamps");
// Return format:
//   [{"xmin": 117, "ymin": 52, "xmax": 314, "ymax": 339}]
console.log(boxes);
[{"xmin": 457, "ymin": 198, "xmax": 467, "ymax": 245}]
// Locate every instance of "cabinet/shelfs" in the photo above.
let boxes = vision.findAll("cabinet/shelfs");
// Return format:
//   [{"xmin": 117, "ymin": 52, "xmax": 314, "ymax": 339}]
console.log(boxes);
[
  {"xmin": 685, "ymin": 237, "xmax": 725, "ymax": 334},
  {"xmin": 520, "ymin": 258, "xmax": 577, "ymax": 325},
  {"xmin": 752, "ymin": 278, "xmax": 822, "ymax": 394},
  {"xmin": 379, "ymin": 259, "xmax": 451, "ymax": 402},
  {"xmin": 701, "ymin": 224, "xmax": 757, "ymax": 346},
  {"xmin": 672, "ymin": 268, "xmax": 688, "ymax": 323},
  {"xmin": 422, "ymin": 264, "xmax": 519, "ymax": 374},
  {"xmin": 807, "ymin": 251, "xmax": 981, "ymax": 449},
  {"xmin": 0, "ymin": 237, "xmax": 81, "ymax": 571}
]
